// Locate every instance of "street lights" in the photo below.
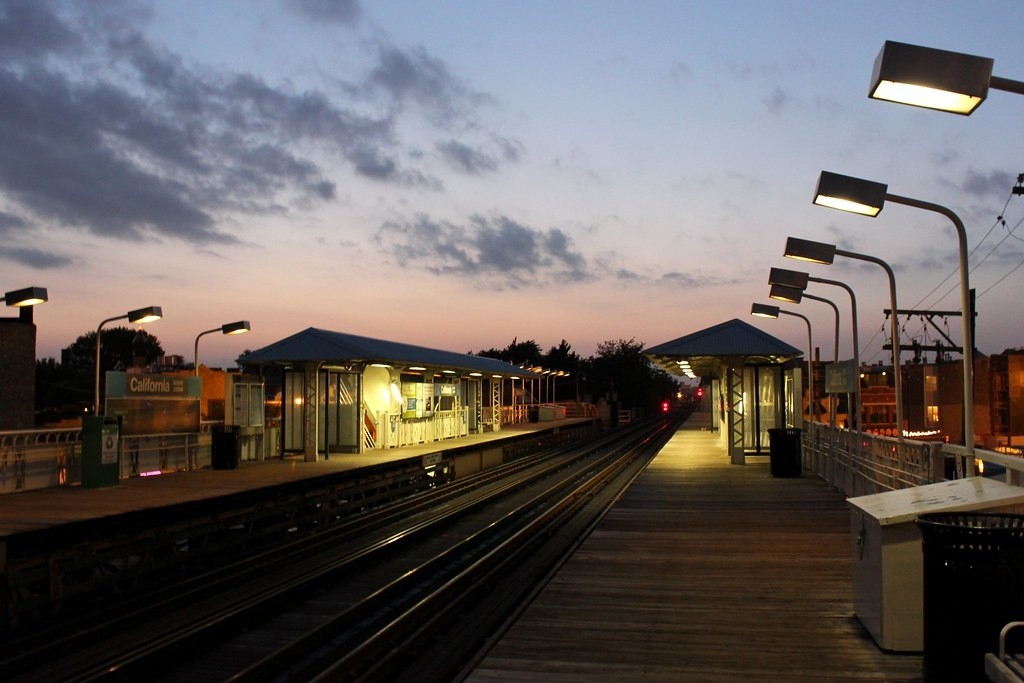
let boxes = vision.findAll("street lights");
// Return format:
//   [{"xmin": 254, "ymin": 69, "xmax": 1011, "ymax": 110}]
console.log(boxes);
[
  {"xmin": 813, "ymin": 170, "xmax": 979, "ymax": 479},
  {"xmin": 193, "ymin": 319, "xmax": 250, "ymax": 378},
  {"xmin": 520, "ymin": 363, "xmax": 570, "ymax": 403},
  {"xmin": 783, "ymin": 237, "xmax": 905, "ymax": 486},
  {"xmin": 768, "ymin": 286, "xmax": 837, "ymax": 450},
  {"xmin": 767, "ymin": 267, "xmax": 864, "ymax": 461},
  {"xmin": 750, "ymin": 302, "xmax": 812, "ymax": 448},
  {"xmin": 95, "ymin": 305, "xmax": 163, "ymax": 418}
]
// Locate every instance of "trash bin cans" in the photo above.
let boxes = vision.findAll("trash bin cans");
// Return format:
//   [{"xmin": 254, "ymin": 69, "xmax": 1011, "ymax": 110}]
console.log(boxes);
[
  {"xmin": 768, "ymin": 428, "xmax": 802, "ymax": 478},
  {"xmin": 211, "ymin": 425, "xmax": 241, "ymax": 471},
  {"xmin": 82, "ymin": 415, "xmax": 121, "ymax": 488},
  {"xmin": 913, "ymin": 510, "xmax": 1024, "ymax": 683},
  {"xmin": 529, "ymin": 407, "xmax": 538, "ymax": 422}
]
